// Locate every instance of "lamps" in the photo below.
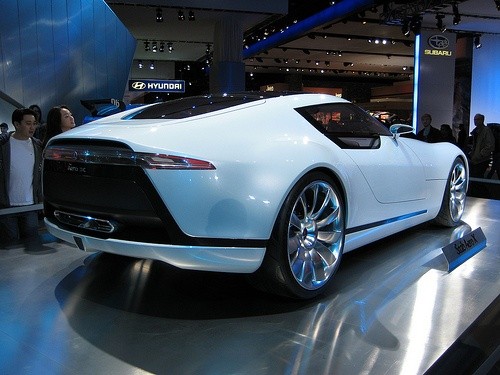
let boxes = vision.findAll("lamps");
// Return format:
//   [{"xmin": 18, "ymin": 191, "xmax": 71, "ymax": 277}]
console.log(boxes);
[{"xmin": 138, "ymin": 0, "xmax": 500, "ymax": 78}]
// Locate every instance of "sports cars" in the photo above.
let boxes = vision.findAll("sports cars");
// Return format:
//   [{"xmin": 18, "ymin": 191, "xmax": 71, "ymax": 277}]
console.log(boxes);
[{"xmin": 40, "ymin": 92, "xmax": 469, "ymax": 300}]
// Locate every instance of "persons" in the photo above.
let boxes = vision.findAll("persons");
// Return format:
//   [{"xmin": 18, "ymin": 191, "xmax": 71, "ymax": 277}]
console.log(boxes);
[
  {"xmin": 418, "ymin": 114, "xmax": 442, "ymax": 143},
  {"xmin": 440, "ymin": 123, "xmax": 467, "ymax": 150},
  {"xmin": 468, "ymin": 113, "xmax": 494, "ymax": 197},
  {"xmin": 386, "ymin": 113, "xmax": 400, "ymax": 127},
  {"xmin": 0, "ymin": 109, "xmax": 44, "ymax": 249},
  {"xmin": 25, "ymin": 105, "xmax": 41, "ymax": 139},
  {"xmin": 43, "ymin": 105, "xmax": 75, "ymax": 148},
  {"xmin": 0, "ymin": 123, "xmax": 11, "ymax": 145}
]
